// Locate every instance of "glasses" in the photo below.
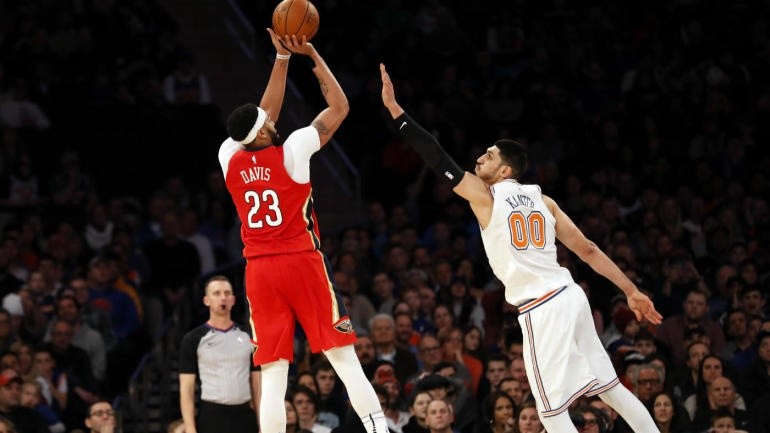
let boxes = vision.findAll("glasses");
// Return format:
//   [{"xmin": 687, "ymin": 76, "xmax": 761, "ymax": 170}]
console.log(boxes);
[
  {"xmin": 636, "ymin": 378, "xmax": 660, "ymax": 385},
  {"xmin": 89, "ymin": 409, "xmax": 114, "ymax": 418},
  {"xmin": 419, "ymin": 345, "xmax": 441, "ymax": 354}
]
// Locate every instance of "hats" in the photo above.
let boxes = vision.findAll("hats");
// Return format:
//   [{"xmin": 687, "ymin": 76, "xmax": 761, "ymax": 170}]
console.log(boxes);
[
  {"xmin": 0, "ymin": 368, "xmax": 26, "ymax": 387},
  {"xmin": 623, "ymin": 351, "xmax": 645, "ymax": 367}
]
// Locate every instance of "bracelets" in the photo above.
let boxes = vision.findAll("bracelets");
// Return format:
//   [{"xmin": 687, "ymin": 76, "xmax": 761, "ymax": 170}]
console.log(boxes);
[{"xmin": 276, "ymin": 52, "xmax": 291, "ymax": 60}]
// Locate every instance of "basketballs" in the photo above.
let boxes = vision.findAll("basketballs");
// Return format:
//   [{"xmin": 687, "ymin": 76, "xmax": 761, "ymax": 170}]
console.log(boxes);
[{"xmin": 272, "ymin": 0, "xmax": 320, "ymax": 44}]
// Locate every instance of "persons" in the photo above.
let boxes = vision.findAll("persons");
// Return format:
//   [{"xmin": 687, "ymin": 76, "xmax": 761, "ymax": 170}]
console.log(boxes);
[
  {"xmin": 217, "ymin": 28, "xmax": 391, "ymax": 433},
  {"xmin": 177, "ymin": 276, "xmax": 262, "ymax": 433},
  {"xmin": 376, "ymin": 63, "xmax": 665, "ymax": 433},
  {"xmin": 0, "ymin": 155, "xmax": 769, "ymax": 433}
]
